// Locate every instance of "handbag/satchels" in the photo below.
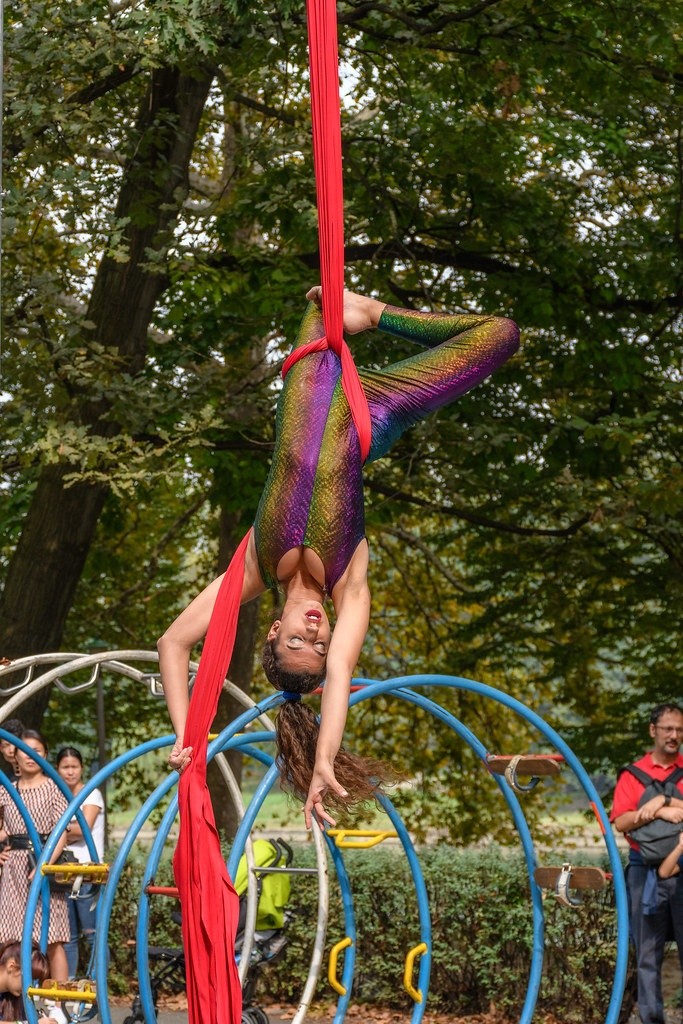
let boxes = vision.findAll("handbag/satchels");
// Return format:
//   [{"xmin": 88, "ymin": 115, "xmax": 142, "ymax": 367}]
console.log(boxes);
[{"xmin": 28, "ymin": 851, "xmax": 78, "ymax": 892}]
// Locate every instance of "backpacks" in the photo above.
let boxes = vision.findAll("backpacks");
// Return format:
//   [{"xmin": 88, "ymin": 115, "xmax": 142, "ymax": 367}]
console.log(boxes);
[{"xmin": 616, "ymin": 765, "xmax": 683, "ymax": 863}]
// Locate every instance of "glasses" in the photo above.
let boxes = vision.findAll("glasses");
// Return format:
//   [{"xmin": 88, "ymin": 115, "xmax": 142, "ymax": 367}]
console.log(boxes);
[{"xmin": 655, "ymin": 726, "xmax": 683, "ymax": 735}]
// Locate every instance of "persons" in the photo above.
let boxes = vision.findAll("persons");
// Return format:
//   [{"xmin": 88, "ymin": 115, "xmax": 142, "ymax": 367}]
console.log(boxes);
[
  {"xmin": 48, "ymin": 746, "xmax": 109, "ymax": 1012},
  {"xmin": 0, "ymin": 716, "xmax": 26, "ymax": 786},
  {"xmin": 153, "ymin": 277, "xmax": 519, "ymax": 836},
  {"xmin": 658, "ymin": 832, "xmax": 683, "ymax": 880},
  {"xmin": 0, "ymin": 937, "xmax": 58, "ymax": 1024},
  {"xmin": 611, "ymin": 702, "xmax": 683, "ymax": 1023},
  {"xmin": 0, "ymin": 728, "xmax": 75, "ymax": 1024}
]
